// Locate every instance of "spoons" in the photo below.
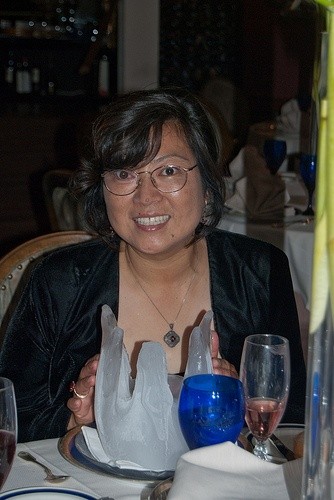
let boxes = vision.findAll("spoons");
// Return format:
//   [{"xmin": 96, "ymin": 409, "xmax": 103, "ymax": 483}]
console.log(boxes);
[{"xmin": 18, "ymin": 450, "xmax": 70, "ymax": 484}]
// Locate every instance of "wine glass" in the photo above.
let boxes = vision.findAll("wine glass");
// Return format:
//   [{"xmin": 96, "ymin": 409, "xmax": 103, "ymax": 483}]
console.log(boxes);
[
  {"xmin": 241, "ymin": 333, "xmax": 293, "ymax": 464},
  {"xmin": 299, "ymin": 154, "xmax": 317, "ymax": 217},
  {"xmin": 263, "ymin": 139, "xmax": 286, "ymax": 175}
]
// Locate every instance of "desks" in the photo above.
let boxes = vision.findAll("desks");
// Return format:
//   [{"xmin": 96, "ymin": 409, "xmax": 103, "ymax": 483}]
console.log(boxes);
[
  {"xmin": 0, "ymin": 425, "xmax": 304, "ymax": 500},
  {"xmin": 212, "ymin": 213, "xmax": 315, "ymax": 370},
  {"xmin": 246, "ymin": 122, "xmax": 310, "ymax": 175}
]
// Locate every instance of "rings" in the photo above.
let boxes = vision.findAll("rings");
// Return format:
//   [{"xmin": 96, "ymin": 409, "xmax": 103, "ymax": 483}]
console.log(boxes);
[{"xmin": 69, "ymin": 381, "xmax": 87, "ymax": 399}]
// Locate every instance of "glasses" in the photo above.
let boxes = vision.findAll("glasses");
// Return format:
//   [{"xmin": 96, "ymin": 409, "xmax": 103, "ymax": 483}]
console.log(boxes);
[{"xmin": 100, "ymin": 163, "xmax": 198, "ymax": 196}]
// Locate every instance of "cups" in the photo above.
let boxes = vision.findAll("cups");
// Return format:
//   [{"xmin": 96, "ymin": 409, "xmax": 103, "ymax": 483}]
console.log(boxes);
[
  {"xmin": 0, "ymin": 377, "xmax": 18, "ymax": 492},
  {"xmin": 179, "ymin": 374, "xmax": 246, "ymax": 454}
]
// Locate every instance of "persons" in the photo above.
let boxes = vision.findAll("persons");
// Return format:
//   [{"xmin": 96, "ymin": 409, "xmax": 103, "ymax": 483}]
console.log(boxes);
[{"xmin": 0, "ymin": 89, "xmax": 307, "ymax": 443}]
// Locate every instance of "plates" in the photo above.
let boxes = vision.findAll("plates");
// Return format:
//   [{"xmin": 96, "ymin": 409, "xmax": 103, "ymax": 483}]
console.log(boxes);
[
  {"xmin": 0, "ymin": 486, "xmax": 101, "ymax": 500},
  {"xmin": 147, "ymin": 476, "xmax": 175, "ymax": 500},
  {"xmin": 244, "ymin": 423, "xmax": 305, "ymax": 465},
  {"xmin": 66, "ymin": 421, "xmax": 173, "ymax": 481}
]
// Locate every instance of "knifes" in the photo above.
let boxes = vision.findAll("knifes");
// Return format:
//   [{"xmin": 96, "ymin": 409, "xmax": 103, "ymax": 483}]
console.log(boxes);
[{"xmin": 270, "ymin": 432, "xmax": 295, "ymax": 462}]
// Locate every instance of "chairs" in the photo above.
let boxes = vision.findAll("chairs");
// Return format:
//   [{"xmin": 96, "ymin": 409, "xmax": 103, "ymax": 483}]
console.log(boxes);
[
  {"xmin": 42, "ymin": 170, "xmax": 94, "ymax": 232},
  {"xmin": 200, "ymin": 76, "xmax": 244, "ymax": 161},
  {"xmin": 0, "ymin": 230, "xmax": 95, "ymax": 333}
]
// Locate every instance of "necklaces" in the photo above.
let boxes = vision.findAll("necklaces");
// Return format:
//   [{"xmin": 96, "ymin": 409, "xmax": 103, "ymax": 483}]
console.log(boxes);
[{"xmin": 125, "ymin": 242, "xmax": 202, "ymax": 347}]
